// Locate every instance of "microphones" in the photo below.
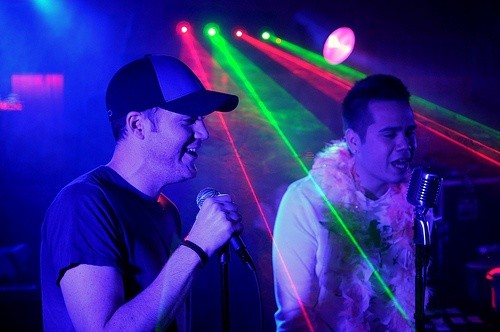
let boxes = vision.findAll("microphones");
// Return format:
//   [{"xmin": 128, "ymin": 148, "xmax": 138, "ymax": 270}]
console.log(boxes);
[
  {"xmin": 407, "ymin": 167, "xmax": 443, "ymax": 210},
  {"xmin": 196, "ymin": 187, "xmax": 256, "ymax": 272}
]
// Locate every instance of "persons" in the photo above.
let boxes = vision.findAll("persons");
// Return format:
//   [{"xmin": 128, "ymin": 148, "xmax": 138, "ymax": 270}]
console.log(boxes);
[
  {"xmin": 39, "ymin": 55, "xmax": 244, "ymax": 332},
  {"xmin": 272, "ymin": 74, "xmax": 433, "ymax": 332}
]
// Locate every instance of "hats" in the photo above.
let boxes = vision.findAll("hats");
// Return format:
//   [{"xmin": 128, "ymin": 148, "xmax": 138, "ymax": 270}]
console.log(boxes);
[{"xmin": 106, "ymin": 52, "xmax": 240, "ymax": 116}]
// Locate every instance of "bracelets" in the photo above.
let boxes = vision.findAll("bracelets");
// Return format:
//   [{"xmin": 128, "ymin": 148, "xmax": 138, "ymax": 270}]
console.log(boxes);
[{"xmin": 180, "ymin": 239, "xmax": 208, "ymax": 269}]
